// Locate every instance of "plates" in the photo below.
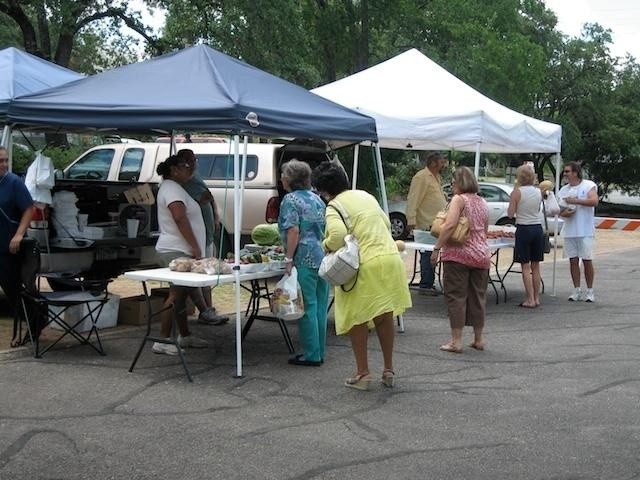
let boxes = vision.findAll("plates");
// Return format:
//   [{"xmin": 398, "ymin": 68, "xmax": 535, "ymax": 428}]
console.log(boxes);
[{"xmin": 225, "ymin": 260, "xmax": 286, "ymax": 273}]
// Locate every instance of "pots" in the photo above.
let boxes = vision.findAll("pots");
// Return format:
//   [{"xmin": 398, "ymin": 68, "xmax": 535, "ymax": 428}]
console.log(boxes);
[
  {"xmin": 46, "ymin": 273, "xmax": 105, "ymax": 291},
  {"xmin": 39, "ymin": 238, "xmax": 95, "ymax": 273}
]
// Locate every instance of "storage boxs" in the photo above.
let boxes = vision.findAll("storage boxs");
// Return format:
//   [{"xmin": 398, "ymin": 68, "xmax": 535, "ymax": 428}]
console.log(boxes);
[
  {"xmin": 119, "ymin": 294, "xmax": 165, "ymax": 325},
  {"xmin": 148, "ymin": 296, "xmax": 166, "ymax": 323},
  {"xmin": 151, "ymin": 286, "xmax": 195, "ymax": 320}
]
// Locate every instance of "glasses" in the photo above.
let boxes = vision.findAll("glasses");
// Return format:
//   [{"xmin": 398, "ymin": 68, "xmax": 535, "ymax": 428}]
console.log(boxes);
[
  {"xmin": 562, "ymin": 169, "xmax": 570, "ymax": 173},
  {"xmin": 193, "ymin": 158, "xmax": 199, "ymax": 163},
  {"xmin": 177, "ymin": 163, "xmax": 191, "ymax": 168}
]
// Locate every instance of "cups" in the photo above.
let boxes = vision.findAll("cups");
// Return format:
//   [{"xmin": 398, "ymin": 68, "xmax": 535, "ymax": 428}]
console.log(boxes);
[
  {"xmin": 78, "ymin": 214, "xmax": 88, "ymax": 232},
  {"xmin": 127, "ymin": 219, "xmax": 140, "ymax": 238}
]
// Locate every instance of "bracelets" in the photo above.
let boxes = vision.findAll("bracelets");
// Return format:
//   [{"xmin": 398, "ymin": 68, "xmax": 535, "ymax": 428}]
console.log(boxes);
[{"xmin": 434, "ymin": 247, "xmax": 440, "ymax": 251}]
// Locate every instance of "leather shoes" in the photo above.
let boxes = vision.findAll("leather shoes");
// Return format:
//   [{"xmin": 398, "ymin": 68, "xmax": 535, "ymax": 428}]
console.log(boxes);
[
  {"xmin": 288, "ymin": 354, "xmax": 325, "ymax": 366},
  {"xmin": 467, "ymin": 343, "xmax": 485, "ymax": 350},
  {"xmin": 440, "ymin": 343, "xmax": 464, "ymax": 353}
]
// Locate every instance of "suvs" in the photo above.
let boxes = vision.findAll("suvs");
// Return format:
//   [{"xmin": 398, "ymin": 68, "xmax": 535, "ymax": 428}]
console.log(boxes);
[{"xmin": 54, "ymin": 142, "xmax": 349, "ymax": 260}]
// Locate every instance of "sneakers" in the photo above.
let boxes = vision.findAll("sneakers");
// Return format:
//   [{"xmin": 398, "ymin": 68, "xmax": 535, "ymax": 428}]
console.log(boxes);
[
  {"xmin": 419, "ymin": 286, "xmax": 440, "ymax": 296},
  {"xmin": 151, "ymin": 337, "xmax": 185, "ymax": 356},
  {"xmin": 198, "ymin": 309, "xmax": 223, "ymax": 325},
  {"xmin": 567, "ymin": 287, "xmax": 583, "ymax": 301},
  {"xmin": 583, "ymin": 288, "xmax": 594, "ymax": 302},
  {"xmin": 177, "ymin": 332, "xmax": 208, "ymax": 348},
  {"xmin": 209, "ymin": 306, "xmax": 229, "ymax": 321}
]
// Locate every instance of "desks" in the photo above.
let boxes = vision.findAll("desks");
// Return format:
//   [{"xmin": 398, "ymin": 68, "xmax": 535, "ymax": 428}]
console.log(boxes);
[
  {"xmin": 404, "ymin": 237, "xmax": 555, "ymax": 304},
  {"xmin": 124, "ymin": 260, "xmax": 295, "ymax": 383}
]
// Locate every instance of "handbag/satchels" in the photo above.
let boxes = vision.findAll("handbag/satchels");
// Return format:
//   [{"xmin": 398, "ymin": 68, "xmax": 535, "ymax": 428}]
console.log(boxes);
[
  {"xmin": 430, "ymin": 194, "xmax": 471, "ymax": 244},
  {"xmin": 537, "ymin": 232, "xmax": 550, "ymax": 253},
  {"xmin": 317, "ymin": 204, "xmax": 360, "ymax": 292}
]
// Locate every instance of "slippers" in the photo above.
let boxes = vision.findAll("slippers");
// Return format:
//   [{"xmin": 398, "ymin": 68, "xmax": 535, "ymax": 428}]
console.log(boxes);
[{"xmin": 519, "ymin": 301, "xmax": 536, "ymax": 308}]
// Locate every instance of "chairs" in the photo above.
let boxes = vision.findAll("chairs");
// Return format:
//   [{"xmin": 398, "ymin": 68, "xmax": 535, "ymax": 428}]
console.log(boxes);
[{"xmin": 0, "ymin": 237, "xmax": 114, "ymax": 359}]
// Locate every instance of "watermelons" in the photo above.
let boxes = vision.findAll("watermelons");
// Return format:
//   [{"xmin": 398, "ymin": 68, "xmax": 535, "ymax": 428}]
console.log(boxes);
[
  {"xmin": 250, "ymin": 223, "xmax": 277, "ymax": 246},
  {"xmin": 271, "ymin": 222, "xmax": 283, "ymax": 246}
]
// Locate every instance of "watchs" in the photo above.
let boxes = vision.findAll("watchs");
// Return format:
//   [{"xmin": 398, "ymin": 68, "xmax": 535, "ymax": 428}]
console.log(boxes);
[{"xmin": 283, "ymin": 257, "xmax": 294, "ymax": 263}]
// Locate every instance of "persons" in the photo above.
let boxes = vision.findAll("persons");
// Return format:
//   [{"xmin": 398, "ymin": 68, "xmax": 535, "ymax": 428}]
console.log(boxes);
[
  {"xmin": 508, "ymin": 165, "xmax": 546, "ymax": 308},
  {"xmin": 310, "ymin": 160, "xmax": 414, "ymax": 391},
  {"xmin": 404, "ymin": 151, "xmax": 448, "ymax": 296},
  {"xmin": 277, "ymin": 158, "xmax": 330, "ymax": 366},
  {"xmin": 429, "ymin": 166, "xmax": 490, "ymax": 352},
  {"xmin": 557, "ymin": 163, "xmax": 599, "ymax": 303},
  {"xmin": 152, "ymin": 155, "xmax": 210, "ymax": 356},
  {"xmin": 0, "ymin": 146, "xmax": 43, "ymax": 342},
  {"xmin": 182, "ymin": 133, "xmax": 193, "ymax": 143},
  {"xmin": 177, "ymin": 149, "xmax": 230, "ymax": 326}
]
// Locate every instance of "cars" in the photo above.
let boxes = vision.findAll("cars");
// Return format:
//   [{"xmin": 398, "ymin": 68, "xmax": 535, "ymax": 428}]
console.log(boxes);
[{"xmin": 386, "ymin": 182, "xmax": 564, "ymax": 241}]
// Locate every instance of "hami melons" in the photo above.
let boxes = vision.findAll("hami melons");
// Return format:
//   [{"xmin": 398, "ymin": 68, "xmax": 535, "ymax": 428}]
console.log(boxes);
[
  {"xmin": 539, "ymin": 181, "xmax": 555, "ymax": 191},
  {"xmin": 395, "ymin": 240, "xmax": 406, "ymax": 251}
]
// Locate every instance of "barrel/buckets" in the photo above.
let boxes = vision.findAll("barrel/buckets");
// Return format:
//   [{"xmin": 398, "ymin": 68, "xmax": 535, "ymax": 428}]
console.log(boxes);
[
  {"xmin": 27, "ymin": 228, "xmax": 50, "ymax": 253},
  {"xmin": 29, "ymin": 204, "xmax": 51, "ymax": 229}
]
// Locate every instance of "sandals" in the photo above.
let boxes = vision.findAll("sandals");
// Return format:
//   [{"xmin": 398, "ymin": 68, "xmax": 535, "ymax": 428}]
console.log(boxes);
[
  {"xmin": 381, "ymin": 369, "xmax": 395, "ymax": 388},
  {"xmin": 344, "ymin": 371, "xmax": 372, "ymax": 392}
]
